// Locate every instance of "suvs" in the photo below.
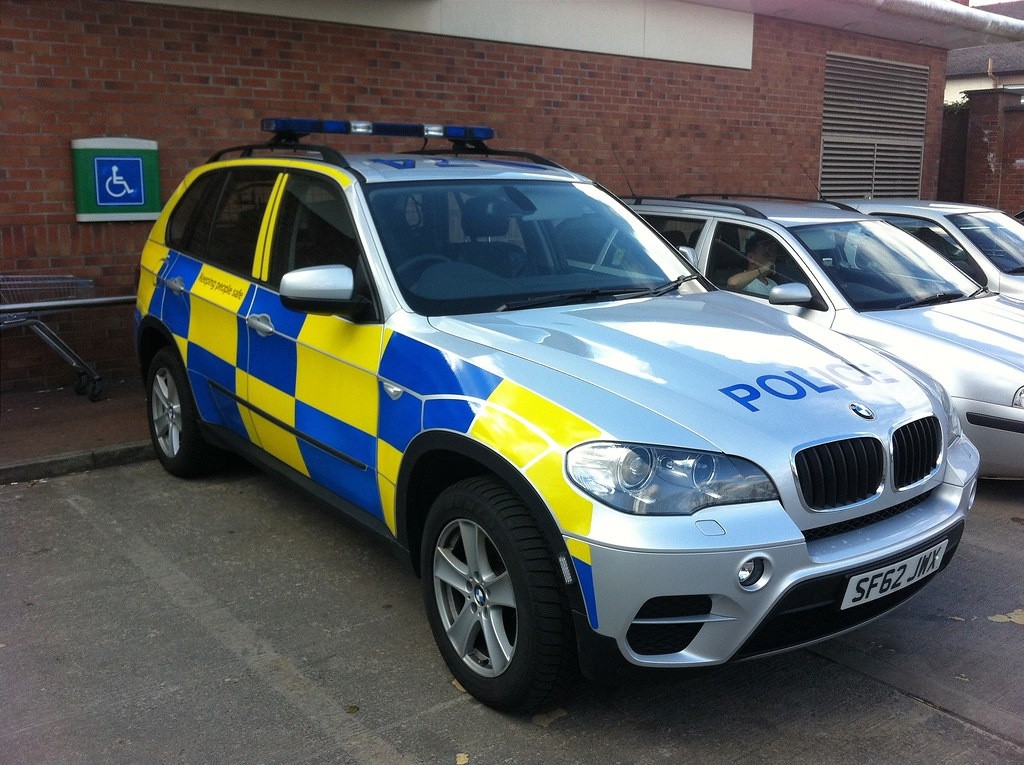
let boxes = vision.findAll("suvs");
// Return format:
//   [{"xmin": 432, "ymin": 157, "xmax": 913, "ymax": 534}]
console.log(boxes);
[{"xmin": 129, "ymin": 115, "xmax": 980, "ymax": 716}]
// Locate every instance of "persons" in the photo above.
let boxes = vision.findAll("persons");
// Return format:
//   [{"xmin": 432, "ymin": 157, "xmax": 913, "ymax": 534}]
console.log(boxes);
[{"xmin": 727, "ymin": 233, "xmax": 777, "ymax": 296}]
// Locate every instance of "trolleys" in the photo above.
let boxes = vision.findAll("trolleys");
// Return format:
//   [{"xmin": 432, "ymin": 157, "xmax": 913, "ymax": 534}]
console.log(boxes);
[{"xmin": 0, "ymin": 276, "xmax": 107, "ymax": 402}]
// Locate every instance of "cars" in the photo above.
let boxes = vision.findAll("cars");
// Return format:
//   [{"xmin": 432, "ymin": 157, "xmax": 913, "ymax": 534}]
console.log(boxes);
[
  {"xmin": 546, "ymin": 192, "xmax": 1024, "ymax": 488},
  {"xmin": 741, "ymin": 196, "xmax": 1024, "ymax": 308}
]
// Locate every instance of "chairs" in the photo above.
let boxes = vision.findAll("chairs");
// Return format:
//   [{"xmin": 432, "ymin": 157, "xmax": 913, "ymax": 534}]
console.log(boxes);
[
  {"xmin": 662, "ymin": 230, "xmax": 701, "ymax": 249},
  {"xmin": 458, "ymin": 198, "xmax": 532, "ymax": 279}
]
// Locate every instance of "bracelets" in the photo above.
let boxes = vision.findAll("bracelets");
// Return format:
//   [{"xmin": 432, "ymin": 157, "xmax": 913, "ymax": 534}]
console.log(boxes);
[{"xmin": 758, "ymin": 268, "xmax": 762, "ymax": 276}]
